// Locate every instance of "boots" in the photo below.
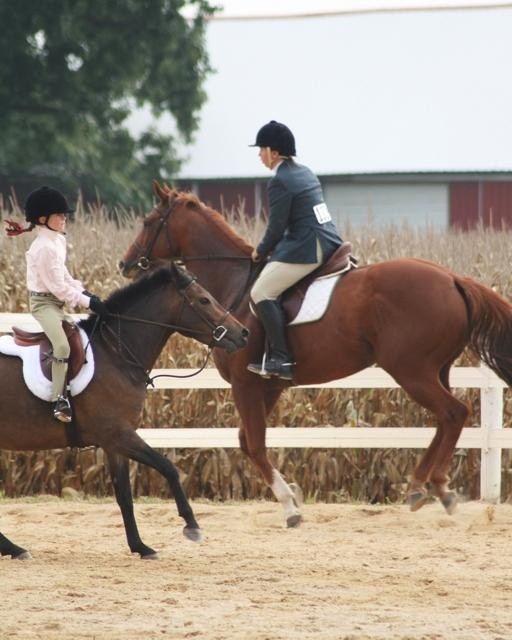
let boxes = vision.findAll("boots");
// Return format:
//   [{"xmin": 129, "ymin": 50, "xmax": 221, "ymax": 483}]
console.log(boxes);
[{"xmin": 246, "ymin": 299, "xmax": 293, "ymax": 380}]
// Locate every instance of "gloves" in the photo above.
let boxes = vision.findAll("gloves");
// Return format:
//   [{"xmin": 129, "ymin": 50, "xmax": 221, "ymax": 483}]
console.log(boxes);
[{"xmin": 83, "ymin": 290, "xmax": 110, "ymax": 321}]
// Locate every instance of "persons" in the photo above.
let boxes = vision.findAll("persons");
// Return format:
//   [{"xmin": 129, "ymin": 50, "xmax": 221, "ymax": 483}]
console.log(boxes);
[
  {"xmin": 248, "ymin": 119, "xmax": 344, "ymax": 383},
  {"xmin": 22, "ymin": 183, "xmax": 108, "ymax": 428}
]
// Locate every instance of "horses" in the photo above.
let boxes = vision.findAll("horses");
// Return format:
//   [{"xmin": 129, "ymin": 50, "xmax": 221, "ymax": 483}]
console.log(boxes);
[
  {"xmin": 118, "ymin": 179, "xmax": 512, "ymax": 529},
  {"xmin": 0, "ymin": 256, "xmax": 251, "ymax": 560}
]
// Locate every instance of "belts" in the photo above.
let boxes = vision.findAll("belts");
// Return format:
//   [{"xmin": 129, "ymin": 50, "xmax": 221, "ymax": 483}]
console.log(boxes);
[{"xmin": 31, "ymin": 292, "xmax": 56, "ymax": 297}]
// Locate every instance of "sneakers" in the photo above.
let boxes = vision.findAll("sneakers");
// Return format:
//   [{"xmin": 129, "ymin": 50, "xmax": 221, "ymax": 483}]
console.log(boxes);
[{"xmin": 54, "ymin": 394, "xmax": 71, "ymax": 422}]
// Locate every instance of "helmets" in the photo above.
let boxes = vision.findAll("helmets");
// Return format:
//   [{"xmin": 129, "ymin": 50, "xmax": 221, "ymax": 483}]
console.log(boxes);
[
  {"xmin": 25, "ymin": 186, "xmax": 75, "ymax": 222},
  {"xmin": 248, "ymin": 120, "xmax": 296, "ymax": 156}
]
